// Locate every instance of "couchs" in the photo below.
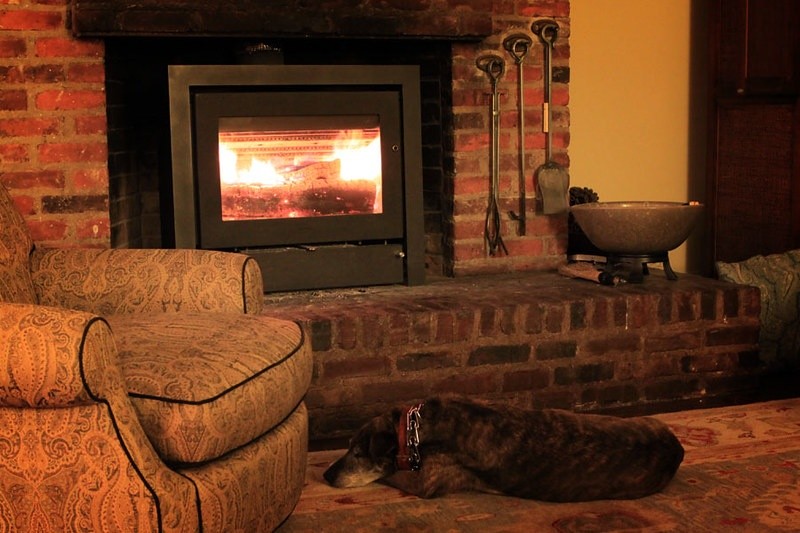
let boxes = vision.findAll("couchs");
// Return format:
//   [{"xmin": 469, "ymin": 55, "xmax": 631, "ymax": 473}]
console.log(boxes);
[{"xmin": 0, "ymin": 182, "xmax": 315, "ymax": 533}]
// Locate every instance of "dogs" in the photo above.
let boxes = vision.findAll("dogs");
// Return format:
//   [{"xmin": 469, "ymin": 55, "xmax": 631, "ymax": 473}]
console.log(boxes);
[{"xmin": 324, "ymin": 398, "xmax": 686, "ymax": 503}]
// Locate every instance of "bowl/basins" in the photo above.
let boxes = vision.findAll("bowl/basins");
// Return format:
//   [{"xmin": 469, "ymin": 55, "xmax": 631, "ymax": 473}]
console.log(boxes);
[{"xmin": 571, "ymin": 201, "xmax": 706, "ymax": 254}]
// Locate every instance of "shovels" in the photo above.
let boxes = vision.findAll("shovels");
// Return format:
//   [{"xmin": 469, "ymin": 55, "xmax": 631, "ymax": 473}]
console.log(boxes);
[{"xmin": 536, "ymin": 21, "xmax": 568, "ymax": 216}]
